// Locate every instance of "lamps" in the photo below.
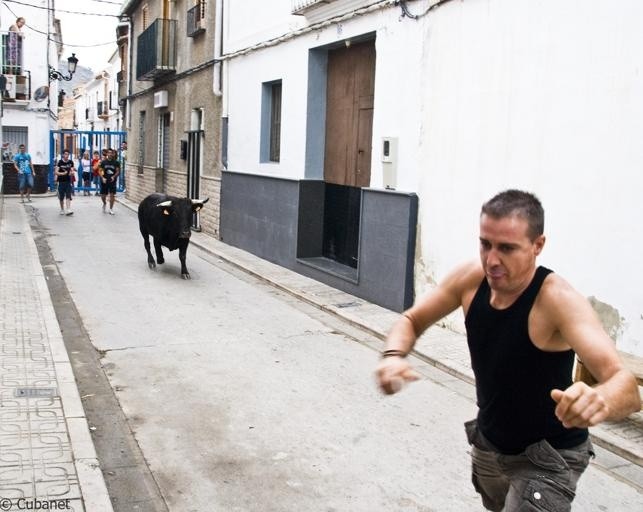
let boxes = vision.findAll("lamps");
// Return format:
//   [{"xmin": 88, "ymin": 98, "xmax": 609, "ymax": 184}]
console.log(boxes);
[{"xmin": 47, "ymin": 52, "xmax": 80, "ymax": 87}]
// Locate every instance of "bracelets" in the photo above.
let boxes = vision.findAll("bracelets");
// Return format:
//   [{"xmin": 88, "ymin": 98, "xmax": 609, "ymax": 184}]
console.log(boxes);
[
  {"xmin": 381, "ymin": 349, "xmax": 406, "ymax": 355},
  {"xmin": 382, "ymin": 353, "xmax": 405, "ymax": 357}
]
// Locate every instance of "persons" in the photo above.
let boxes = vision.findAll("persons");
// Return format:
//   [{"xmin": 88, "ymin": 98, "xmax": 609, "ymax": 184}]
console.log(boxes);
[
  {"xmin": 97, "ymin": 149, "xmax": 119, "ymax": 215},
  {"xmin": 6, "ymin": 16, "xmax": 26, "ymax": 75},
  {"xmin": 374, "ymin": 188, "xmax": 643, "ymax": 512},
  {"xmin": 54, "ymin": 149, "xmax": 74, "ymax": 216},
  {"xmin": 11, "ymin": 144, "xmax": 35, "ymax": 204},
  {"xmin": 53, "ymin": 141, "xmax": 127, "ymax": 201}
]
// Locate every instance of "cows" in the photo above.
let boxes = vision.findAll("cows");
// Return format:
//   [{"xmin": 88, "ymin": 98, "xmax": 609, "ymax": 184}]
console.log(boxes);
[{"xmin": 137, "ymin": 192, "xmax": 209, "ymax": 281}]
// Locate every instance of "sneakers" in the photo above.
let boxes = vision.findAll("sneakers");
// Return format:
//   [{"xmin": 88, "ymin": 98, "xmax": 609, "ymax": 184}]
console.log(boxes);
[
  {"xmin": 84, "ymin": 192, "xmax": 101, "ymax": 196},
  {"xmin": 60, "ymin": 211, "xmax": 65, "ymax": 215},
  {"xmin": 103, "ymin": 204, "xmax": 115, "ymax": 215},
  {"xmin": 20, "ymin": 194, "xmax": 32, "ymax": 203},
  {"xmin": 66, "ymin": 210, "xmax": 73, "ymax": 215}
]
